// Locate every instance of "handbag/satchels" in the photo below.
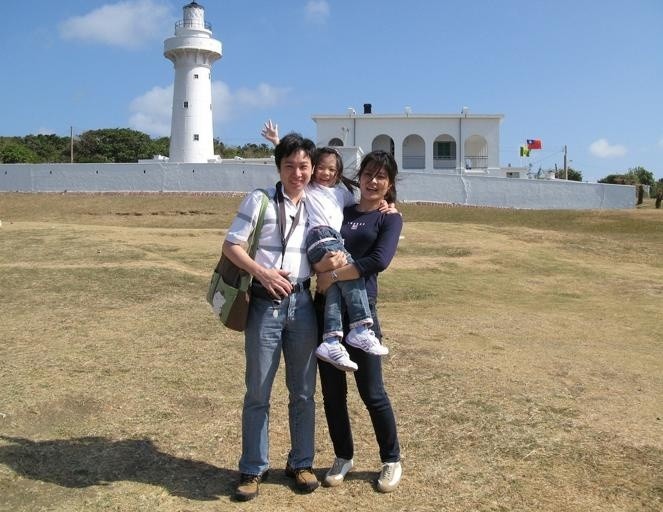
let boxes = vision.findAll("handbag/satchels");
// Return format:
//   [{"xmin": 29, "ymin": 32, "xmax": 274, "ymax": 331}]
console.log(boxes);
[{"xmin": 206, "ymin": 189, "xmax": 269, "ymax": 332}]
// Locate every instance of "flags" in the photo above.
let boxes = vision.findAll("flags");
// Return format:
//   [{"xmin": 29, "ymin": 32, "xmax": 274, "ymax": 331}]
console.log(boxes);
[
  {"xmin": 520, "ymin": 146, "xmax": 531, "ymax": 157},
  {"xmin": 527, "ymin": 138, "xmax": 542, "ymax": 150}
]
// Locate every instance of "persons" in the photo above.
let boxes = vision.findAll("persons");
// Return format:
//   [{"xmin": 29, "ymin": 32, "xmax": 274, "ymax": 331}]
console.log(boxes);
[
  {"xmin": 259, "ymin": 116, "xmax": 389, "ymax": 373},
  {"xmin": 221, "ymin": 130, "xmax": 402, "ymax": 502},
  {"xmin": 311, "ymin": 148, "xmax": 403, "ymax": 493}
]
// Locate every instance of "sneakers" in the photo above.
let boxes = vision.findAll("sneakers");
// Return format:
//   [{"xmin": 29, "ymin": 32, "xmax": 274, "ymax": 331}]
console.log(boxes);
[
  {"xmin": 377, "ymin": 461, "xmax": 404, "ymax": 493},
  {"xmin": 236, "ymin": 473, "xmax": 261, "ymax": 501},
  {"xmin": 285, "ymin": 450, "xmax": 318, "ymax": 493},
  {"xmin": 324, "ymin": 456, "xmax": 353, "ymax": 487},
  {"xmin": 315, "ymin": 342, "xmax": 358, "ymax": 371},
  {"xmin": 345, "ymin": 328, "xmax": 389, "ymax": 356}
]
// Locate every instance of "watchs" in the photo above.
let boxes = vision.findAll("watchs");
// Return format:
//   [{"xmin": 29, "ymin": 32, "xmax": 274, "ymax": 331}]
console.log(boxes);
[{"xmin": 328, "ymin": 269, "xmax": 340, "ymax": 283}]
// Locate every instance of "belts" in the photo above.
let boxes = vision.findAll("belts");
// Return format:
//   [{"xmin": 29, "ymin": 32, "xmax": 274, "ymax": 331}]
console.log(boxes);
[{"xmin": 252, "ymin": 278, "xmax": 311, "ymax": 299}]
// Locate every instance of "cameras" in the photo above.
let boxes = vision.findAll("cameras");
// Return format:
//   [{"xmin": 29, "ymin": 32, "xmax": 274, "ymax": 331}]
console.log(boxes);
[{"xmin": 268, "ymin": 276, "xmax": 291, "ymax": 305}]
